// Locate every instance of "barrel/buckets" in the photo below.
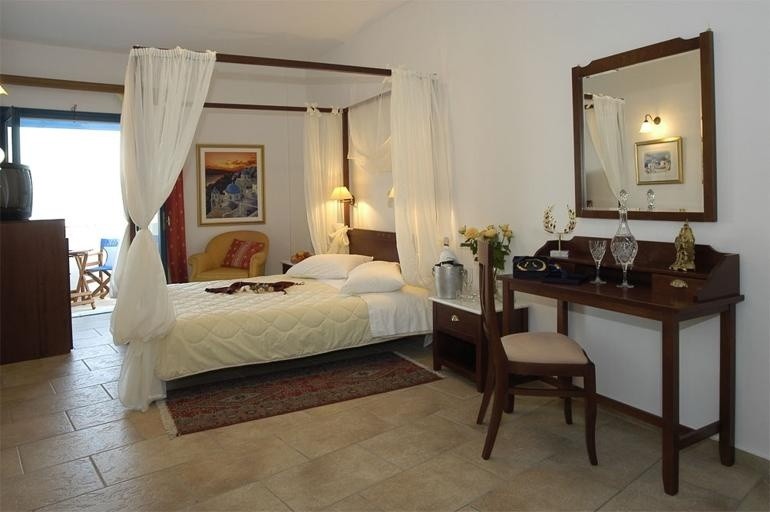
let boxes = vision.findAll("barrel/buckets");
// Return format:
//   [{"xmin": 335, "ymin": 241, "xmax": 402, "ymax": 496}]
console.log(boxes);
[{"xmin": 431, "ymin": 264, "xmax": 465, "ymax": 298}]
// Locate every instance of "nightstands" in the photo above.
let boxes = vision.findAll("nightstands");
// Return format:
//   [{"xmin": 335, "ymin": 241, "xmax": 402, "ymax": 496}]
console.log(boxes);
[
  {"xmin": 280, "ymin": 260, "xmax": 294, "ymax": 274},
  {"xmin": 428, "ymin": 296, "xmax": 528, "ymax": 394}
]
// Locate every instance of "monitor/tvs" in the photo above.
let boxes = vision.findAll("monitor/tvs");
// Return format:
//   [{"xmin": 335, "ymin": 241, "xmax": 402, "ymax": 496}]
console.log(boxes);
[{"xmin": 0, "ymin": 162, "xmax": 33, "ymax": 219}]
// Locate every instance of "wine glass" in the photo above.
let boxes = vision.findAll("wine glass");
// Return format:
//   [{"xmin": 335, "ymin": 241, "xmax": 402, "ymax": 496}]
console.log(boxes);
[
  {"xmin": 615, "ymin": 241, "xmax": 638, "ymax": 290},
  {"xmin": 586, "ymin": 238, "xmax": 608, "ymax": 285},
  {"xmin": 461, "ymin": 268, "xmax": 475, "ymax": 295}
]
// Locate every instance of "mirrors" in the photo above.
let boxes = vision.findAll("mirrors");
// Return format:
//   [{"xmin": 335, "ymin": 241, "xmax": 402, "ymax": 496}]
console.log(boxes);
[{"xmin": 571, "ymin": 30, "xmax": 717, "ymax": 222}]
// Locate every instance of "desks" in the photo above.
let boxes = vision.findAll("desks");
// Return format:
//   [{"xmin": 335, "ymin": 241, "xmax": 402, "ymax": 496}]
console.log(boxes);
[
  {"xmin": 68, "ymin": 249, "xmax": 96, "ymax": 310},
  {"xmin": 496, "ymin": 235, "xmax": 745, "ymax": 495}
]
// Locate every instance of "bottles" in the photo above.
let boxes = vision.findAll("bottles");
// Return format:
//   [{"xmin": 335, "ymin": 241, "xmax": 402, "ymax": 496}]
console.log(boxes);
[
  {"xmin": 440, "ymin": 237, "xmax": 457, "ymax": 266},
  {"xmin": 610, "ymin": 187, "xmax": 639, "ymax": 265},
  {"xmin": 645, "ymin": 188, "xmax": 657, "ymax": 210}
]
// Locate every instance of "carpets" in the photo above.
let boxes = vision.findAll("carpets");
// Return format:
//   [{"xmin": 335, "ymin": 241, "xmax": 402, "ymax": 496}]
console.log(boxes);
[{"xmin": 155, "ymin": 350, "xmax": 448, "ymax": 440}]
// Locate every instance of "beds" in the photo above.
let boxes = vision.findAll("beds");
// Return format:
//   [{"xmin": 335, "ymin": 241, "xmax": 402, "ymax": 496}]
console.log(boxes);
[{"xmin": 128, "ymin": 46, "xmax": 441, "ymax": 405}]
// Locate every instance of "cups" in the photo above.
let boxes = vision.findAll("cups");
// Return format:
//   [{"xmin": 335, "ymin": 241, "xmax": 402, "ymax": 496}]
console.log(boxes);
[{"xmin": 455, "ymin": 288, "xmax": 482, "ymax": 308}]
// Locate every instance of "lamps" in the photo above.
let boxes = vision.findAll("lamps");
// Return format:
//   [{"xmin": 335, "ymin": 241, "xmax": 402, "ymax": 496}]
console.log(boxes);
[
  {"xmin": 330, "ymin": 187, "xmax": 355, "ymax": 205},
  {"xmin": 639, "ymin": 114, "xmax": 660, "ymax": 134}
]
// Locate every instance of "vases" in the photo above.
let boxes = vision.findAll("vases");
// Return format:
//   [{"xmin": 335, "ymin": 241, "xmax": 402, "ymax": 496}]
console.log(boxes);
[{"xmin": 493, "ymin": 277, "xmax": 498, "ymax": 294}]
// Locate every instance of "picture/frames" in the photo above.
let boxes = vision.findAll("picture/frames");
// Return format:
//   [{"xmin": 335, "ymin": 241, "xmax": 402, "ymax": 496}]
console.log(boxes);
[
  {"xmin": 634, "ymin": 136, "xmax": 684, "ymax": 185},
  {"xmin": 196, "ymin": 143, "xmax": 266, "ymax": 226}
]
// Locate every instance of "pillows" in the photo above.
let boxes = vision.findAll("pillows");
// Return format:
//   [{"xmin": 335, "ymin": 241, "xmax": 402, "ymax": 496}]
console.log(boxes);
[
  {"xmin": 284, "ymin": 254, "xmax": 374, "ymax": 279},
  {"xmin": 340, "ymin": 261, "xmax": 406, "ymax": 296},
  {"xmin": 221, "ymin": 238, "xmax": 265, "ymax": 269}
]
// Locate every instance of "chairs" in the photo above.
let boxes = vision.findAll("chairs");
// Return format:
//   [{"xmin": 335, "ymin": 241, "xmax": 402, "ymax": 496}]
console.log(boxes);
[
  {"xmin": 188, "ymin": 230, "xmax": 269, "ymax": 281},
  {"xmin": 476, "ymin": 239, "xmax": 598, "ymax": 466},
  {"xmin": 83, "ymin": 238, "xmax": 119, "ymax": 297}
]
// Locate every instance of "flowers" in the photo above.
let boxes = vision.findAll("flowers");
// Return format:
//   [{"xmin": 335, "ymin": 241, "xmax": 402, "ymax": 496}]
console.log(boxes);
[{"xmin": 458, "ymin": 225, "xmax": 513, "ymax": 275}]
